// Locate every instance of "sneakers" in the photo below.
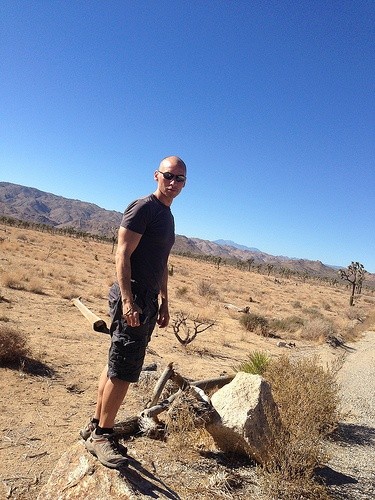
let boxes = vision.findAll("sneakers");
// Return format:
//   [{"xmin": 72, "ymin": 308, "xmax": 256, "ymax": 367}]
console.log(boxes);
[
  {"xmin": 84, "ymin": 428, "xmax": 128, "ymax": 468},
  {"xmin": 79, "ymin": 418, "xmax": 127, "ymax": 457}
]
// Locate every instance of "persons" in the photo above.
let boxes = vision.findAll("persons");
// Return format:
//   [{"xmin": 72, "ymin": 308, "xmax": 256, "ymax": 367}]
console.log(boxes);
[{"xmin": 80, "ymin": 156, "xmax": 187, "ymax": 467}]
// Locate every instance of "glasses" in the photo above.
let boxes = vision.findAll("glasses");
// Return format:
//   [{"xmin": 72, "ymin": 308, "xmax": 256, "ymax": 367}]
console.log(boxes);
[{"xmin": 158, "ymin": 171, "xmax": 186, "ymax": 182}]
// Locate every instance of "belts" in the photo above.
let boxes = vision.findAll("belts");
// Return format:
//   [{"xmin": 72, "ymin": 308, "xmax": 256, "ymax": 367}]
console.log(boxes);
[{"xmin": 132, "ymin": 287, "xmax": 158, "ymax": 300}]
